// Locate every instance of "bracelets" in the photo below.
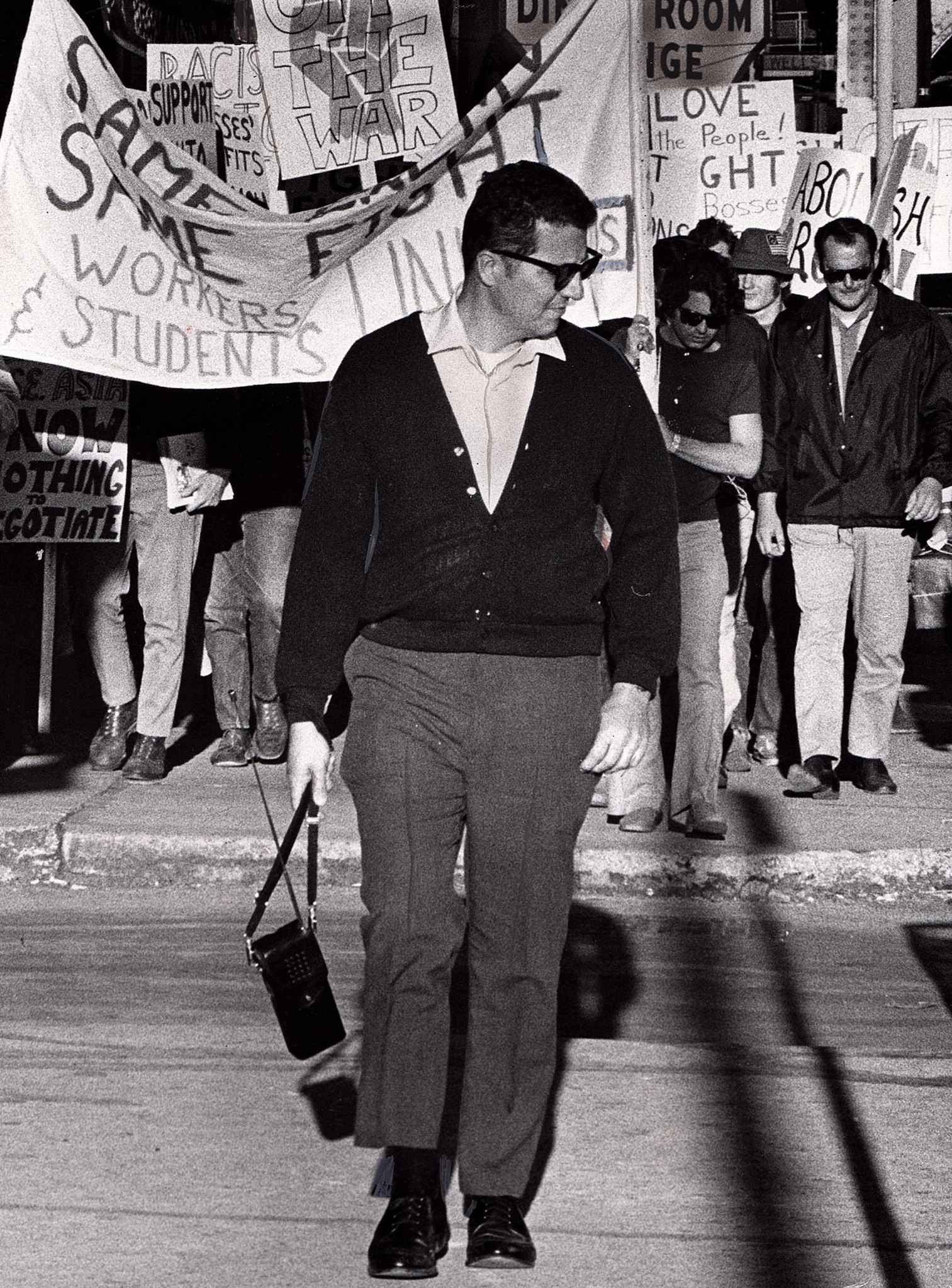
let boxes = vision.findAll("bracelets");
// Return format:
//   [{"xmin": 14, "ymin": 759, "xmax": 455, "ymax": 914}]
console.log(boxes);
[{"xmin": 669, "ymin": 432, "xmax": 681, "ymax": 454}]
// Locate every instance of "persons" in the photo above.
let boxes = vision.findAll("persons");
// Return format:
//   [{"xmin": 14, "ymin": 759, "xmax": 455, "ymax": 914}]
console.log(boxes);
[
  {"xmin": 276, "ymin": 157, "xmax": 675, "ymax": 1278},
  {"xmin": 2, "ymin": 216, "xmax": 952, "ymax": 840}
]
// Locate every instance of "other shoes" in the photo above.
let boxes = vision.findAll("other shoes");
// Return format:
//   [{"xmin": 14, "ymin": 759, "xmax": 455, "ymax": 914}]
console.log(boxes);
[
  {"xmin": 619, "ymin": 808, "xmax": 661, "ymax": 832},
  {"xmin": 686, "ymin": 802, "xmax": 728, "ymax": 835},
  {"xmin": 754, "ymin": 738, "xmax": 780, "ymax": 765},
  {"xmin": 724, "ymin": 747, "xmax": 751, "ymax": 771}
]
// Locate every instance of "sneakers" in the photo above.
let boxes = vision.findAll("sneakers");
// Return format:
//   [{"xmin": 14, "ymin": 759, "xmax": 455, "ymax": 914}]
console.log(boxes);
[
  {"xmin": 252, "ymin": 699, "xmax": 288, "ymax": 759},
  {"xmin": 121, "ymin": 735, "xmax": 166, "ymax": 778},
  {"xmin": 88, "ymin": 697, "xmax": 138, "ymax": 770},
  {"xmin": 210, "ymin": 727, "xmax": 255, "ymax": 768}
]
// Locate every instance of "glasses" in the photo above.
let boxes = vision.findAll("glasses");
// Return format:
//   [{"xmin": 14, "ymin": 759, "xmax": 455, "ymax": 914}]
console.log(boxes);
[
  {"xmin": 824, "ymin": 261, "xmax": 873, "ymax": 283},
  {"xmin": 490, "ymin": 247, "xmax": 603, "ymax": 290},
  {"xmin": 679, "ymin": 307, "xmax": 725, "ymax": 330}
]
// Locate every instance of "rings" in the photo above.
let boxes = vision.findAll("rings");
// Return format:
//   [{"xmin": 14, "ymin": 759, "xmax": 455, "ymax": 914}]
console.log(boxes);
[{"xmin": 637, "ymin": 341, "xmax": 644, "ymax": 351}]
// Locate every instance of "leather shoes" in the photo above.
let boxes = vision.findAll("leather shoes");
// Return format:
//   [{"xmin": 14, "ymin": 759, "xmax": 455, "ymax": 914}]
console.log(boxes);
[
  {"xmin": 368, "ymin": 1194, "xmax": 451, "ymax": 1279},
  {"xmin": 464, "ymin": 1198, "xmax": 536, "ymax": 1269},
  {"xmin": 845, "ymin": 753, "xmax": 897, "ymax": 795},
  {"xmin": 786, "ymin": 760, "xmax": 842, "ymax": 798}
]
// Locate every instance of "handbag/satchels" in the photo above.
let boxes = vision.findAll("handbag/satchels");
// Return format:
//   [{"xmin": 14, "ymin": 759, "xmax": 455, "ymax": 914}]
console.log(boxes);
[{"xmin": 250, "ymin": 917, "xmax": 346, "ymax": 1061}]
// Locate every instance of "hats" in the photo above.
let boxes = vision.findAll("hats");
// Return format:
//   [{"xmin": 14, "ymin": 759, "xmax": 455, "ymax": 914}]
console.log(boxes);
[{"xmin": 732, "ymin": 229, "xmax": 803, "ymax": 275}]
